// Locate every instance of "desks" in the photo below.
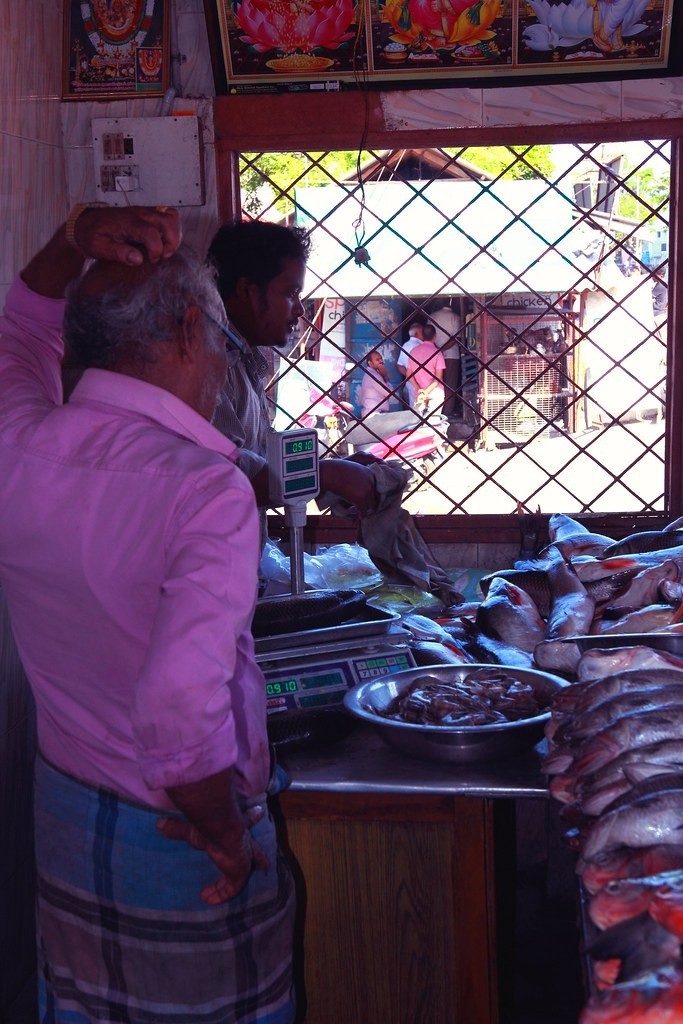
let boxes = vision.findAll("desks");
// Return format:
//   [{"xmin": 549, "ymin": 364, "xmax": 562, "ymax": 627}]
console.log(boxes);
[{"xmin": 270, "ymin": 719, "xmax": 550, "ymax": 1024}]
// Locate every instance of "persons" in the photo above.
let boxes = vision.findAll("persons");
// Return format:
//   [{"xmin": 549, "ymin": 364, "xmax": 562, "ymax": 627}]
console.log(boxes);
[
  {"xmin": 0, "ymin": 201, "xmax": 298, "ymax": 1024},
  {"xmin": 204, "ymin": 218, "xmax": 385, "ymax": 561},
  {"xmin": 361, "ymin": 351, "xmax": 395, "ymax": 419},
  {"xmin": 397, "ymin": 297, "xmax": 461, "ymax": 415},
  {"xmin": 651, "ymin": 264, "xmax": 668, "ymax": 365},
  {"xmin": 505, "ymin": 327, "xmax": 564, "ymax": 355}
]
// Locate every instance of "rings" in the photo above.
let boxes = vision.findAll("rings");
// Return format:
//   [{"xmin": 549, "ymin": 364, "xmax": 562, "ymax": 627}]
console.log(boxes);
[{"xmin": 156, "ymin": 205, "xmax": 168, "ymax": 213}]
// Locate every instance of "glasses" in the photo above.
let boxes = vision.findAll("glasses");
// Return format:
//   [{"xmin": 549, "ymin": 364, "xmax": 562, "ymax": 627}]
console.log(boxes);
[{"xmin": 178, "ymin": 302, "xmax": 246, "ymax": 369}]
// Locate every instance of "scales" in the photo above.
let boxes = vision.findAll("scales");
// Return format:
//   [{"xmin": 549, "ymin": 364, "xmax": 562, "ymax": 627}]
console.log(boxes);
[{"xmin": 250, "ymin": 427, "xmax": 419, "ymax": 724}]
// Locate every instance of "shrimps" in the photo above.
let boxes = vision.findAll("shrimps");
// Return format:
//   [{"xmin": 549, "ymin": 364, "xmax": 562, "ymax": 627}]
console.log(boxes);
[{"xmin": 362, "ymin": 666, "xmax": 558, "ymax": 727}]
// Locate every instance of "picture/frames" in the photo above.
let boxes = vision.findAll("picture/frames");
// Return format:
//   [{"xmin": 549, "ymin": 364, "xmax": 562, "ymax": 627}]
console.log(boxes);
[
  {"xmin": 203, "ymin": 0, "xmax": 683, "ymax": 96},
  {"xmin": 61, "ymin": 0, "xmax": 171, "ymax": 103}
]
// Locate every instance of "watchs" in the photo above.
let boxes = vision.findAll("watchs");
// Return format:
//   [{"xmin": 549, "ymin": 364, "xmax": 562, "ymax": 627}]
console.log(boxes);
[{"xmin": 65, "ymin": 201, "xmax": 110, "ymax": 255}]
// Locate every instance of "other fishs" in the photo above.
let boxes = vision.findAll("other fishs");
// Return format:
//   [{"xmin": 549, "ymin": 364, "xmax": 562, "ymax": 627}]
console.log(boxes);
[
  {"xmin": 538, "ymin": 667, "xmax": 683, "ymax": 1024},
  {"xmin": 401, "ymin": 514, "xmax": 683, "ymax": 683}
]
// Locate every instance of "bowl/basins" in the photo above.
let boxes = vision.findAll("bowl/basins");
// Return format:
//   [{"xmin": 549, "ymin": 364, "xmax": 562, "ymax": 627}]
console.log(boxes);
[
  {"xmin": 341, "ymin": 662, "xmax": 575, "ymax": 768},
  {"xmin": 498, "ymin": 344, "xmax": 517, "ymax": 354},
  {"xmin": 560, "ymin": 633, "xmax": 683, "ymax": 659}
]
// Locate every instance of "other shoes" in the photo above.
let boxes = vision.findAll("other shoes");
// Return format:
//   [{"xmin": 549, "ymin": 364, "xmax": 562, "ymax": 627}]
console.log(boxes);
[{"xmin": 444, "ymin": 410, "xmax": 457, "ymax": 418}]
